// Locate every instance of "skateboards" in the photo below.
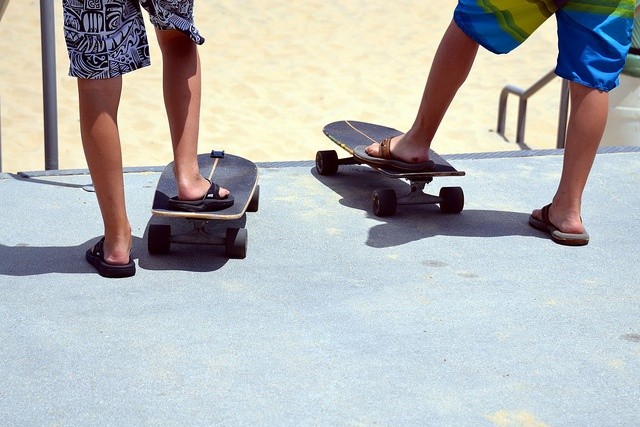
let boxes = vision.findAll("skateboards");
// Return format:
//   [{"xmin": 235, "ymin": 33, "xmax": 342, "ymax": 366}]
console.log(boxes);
[
  {"xmin": 315, "ymin": 120, "xmax": 466, "ymax": 216},
  {"xmin": 148, "ymin": 150, "xmax": 260, "ymax": 258}
]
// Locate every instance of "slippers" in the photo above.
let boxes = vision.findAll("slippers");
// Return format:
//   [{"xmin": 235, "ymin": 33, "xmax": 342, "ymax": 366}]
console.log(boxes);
[
  {"xmin": 85, "ymin": 237, "xmax": 136, "ymax": 278},
  {"xmin": 353, "ymin": 135, "xmax": 435, "ymax": 172},
  {"xmin": 528, "ymin": 203, "xmax": 589, "ymax": 247},
  {"xmin": 168, "ymin": 179, "xmax": 234, "ymax": 212}
]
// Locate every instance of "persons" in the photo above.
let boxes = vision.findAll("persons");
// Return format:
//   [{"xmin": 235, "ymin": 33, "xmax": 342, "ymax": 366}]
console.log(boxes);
[
  {"xmin": 62, "ymin": 0, "xmax": 235, "ymax": 277},
  {"xmin": 352, "ymin": 1, "xmax": 640, "ymax": 246}
]
